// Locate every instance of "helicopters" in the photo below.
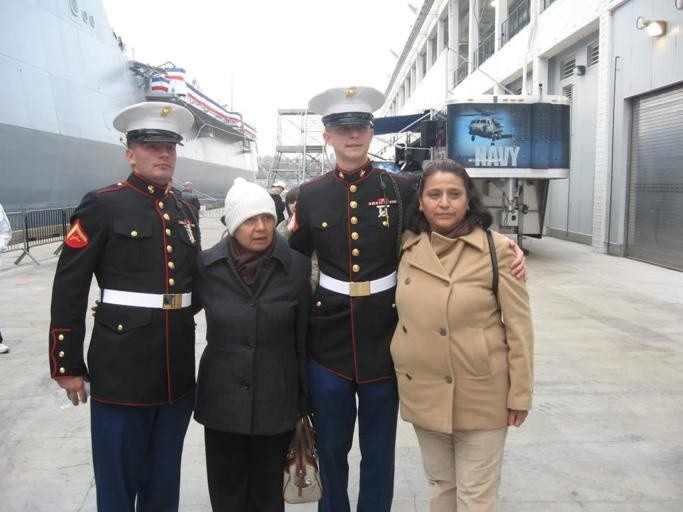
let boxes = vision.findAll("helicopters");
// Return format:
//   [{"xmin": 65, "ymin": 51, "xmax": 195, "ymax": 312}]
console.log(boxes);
[{"xmin": 457, "ymin": 107, "xmax": 512, "ymax": 146}]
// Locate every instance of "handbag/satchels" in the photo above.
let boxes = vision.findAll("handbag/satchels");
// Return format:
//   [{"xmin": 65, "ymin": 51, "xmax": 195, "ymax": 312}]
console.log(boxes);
[{"xmin": 282, "ymin": 415, "xmax": 324, "ymax": 504}]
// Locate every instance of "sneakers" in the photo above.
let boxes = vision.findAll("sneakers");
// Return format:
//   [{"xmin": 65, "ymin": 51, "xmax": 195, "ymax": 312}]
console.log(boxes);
[{"xmin": 0, "ymin": 344, "xmax": 10, "ymax": 353}]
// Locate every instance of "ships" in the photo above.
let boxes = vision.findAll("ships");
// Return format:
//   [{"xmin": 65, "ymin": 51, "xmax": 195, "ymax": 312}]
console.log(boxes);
[{"xmin": 0, "ymin": 0, "xmax": 259, "ymax": 247}]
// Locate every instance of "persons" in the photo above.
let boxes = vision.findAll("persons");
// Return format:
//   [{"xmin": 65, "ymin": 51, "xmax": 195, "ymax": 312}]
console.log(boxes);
[
  {"xmin": 181, "ymin": 182, "xmax": 201, "ymax": 211},
  {"xmin": 48, "ymin": 101, "xmax": 201, "ymax": 511},
  {"xmin": 287, "ymin": 87, "xmax": 528, "ymax": 512},
  {"xmin": 269, "ymin": 181, "xmax": 320, "ymax": 294},
  {"xmin": 92, "ymin": 177, "xmax": 311, "ymax": 512},
  {"xmin": 390, "ymin": 159, "xmax": 533, "ymax": 512},
  {"xmin": 0, "ymin": 203, "xmax": 10, "ymax": 355}
]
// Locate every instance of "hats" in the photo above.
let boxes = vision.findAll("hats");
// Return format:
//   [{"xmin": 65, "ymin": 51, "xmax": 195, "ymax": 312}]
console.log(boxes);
[
  {"xmin": 224, "ymin": 177, "xmax": 277, "ymax": 237},
  {"xmin": 112, "ymin": 101, "xmax": 194, "ymax": 146},
  {"xmin": 272, "ymin": 181, "xmax": 287, "ymax": 190},
  {"xmin": 308, "ymin": 86, "xmax": 386, "ymax": 127}
]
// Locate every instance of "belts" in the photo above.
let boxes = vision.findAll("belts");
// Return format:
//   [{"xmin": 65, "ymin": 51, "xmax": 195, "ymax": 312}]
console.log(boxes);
[
  {"xmin": 102, "ymin": 288, "xmax": 192, "ymax": 309},
  {"xmin": 319, "ymin": 270, "xmax": 397, "ymax": 297}
]
